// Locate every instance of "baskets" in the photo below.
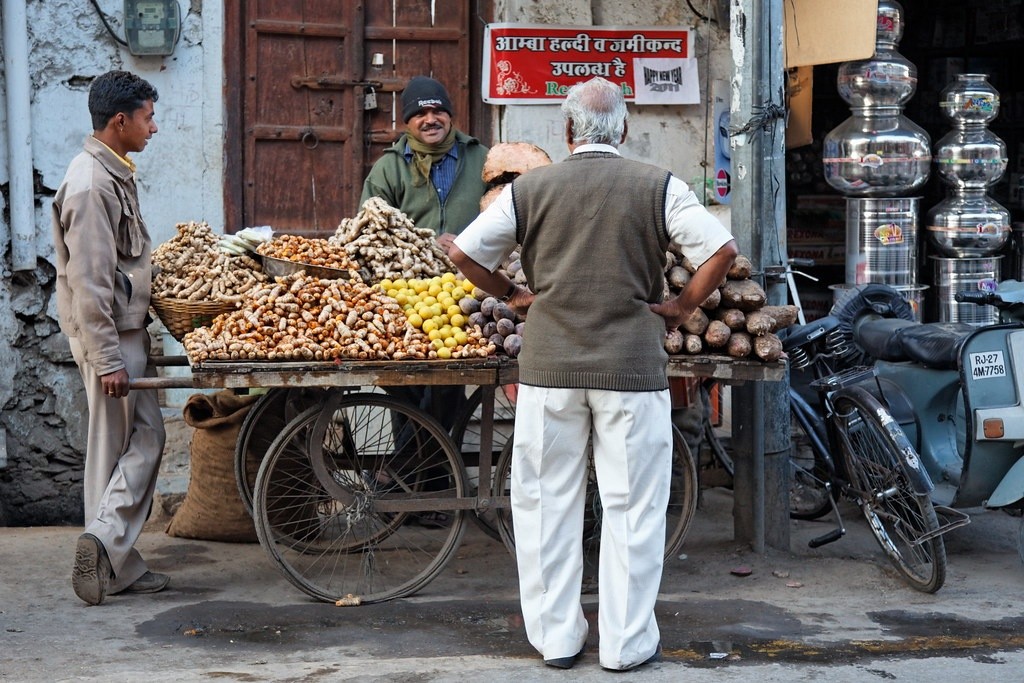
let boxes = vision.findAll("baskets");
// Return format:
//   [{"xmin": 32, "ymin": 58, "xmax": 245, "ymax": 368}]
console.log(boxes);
[{"xmin": 150, "ymin": 295, "xmax": 239, "ymax": 342}]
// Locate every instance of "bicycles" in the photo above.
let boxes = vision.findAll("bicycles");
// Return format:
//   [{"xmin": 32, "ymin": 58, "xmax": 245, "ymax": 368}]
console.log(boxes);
[{"xmin": 691, "ymin": 256, "xmax": 976, "ymax": 594}]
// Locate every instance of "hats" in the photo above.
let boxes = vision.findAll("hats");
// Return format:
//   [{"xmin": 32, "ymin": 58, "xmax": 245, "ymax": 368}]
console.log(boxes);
[{"xmin": 399, "ymin": 76, "xmax": 453, "ymax": 124}]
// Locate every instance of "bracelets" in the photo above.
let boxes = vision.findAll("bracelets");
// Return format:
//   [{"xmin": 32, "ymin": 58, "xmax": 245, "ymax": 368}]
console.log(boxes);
[{"xmin": 499, "ymin": 285, "xmax": 516, "ymax": 301}]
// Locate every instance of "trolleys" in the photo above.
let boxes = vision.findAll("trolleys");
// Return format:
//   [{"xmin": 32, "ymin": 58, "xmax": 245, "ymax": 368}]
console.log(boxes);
[{"xmin": 118, "ymin": 350, "xmax": 788, "ymax": 605}]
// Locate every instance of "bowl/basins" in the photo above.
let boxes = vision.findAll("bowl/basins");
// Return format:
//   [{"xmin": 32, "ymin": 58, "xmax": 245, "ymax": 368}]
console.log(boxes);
[{"xmin": 252, "ymin": 249, "xmax": 349, "ymax": 279}]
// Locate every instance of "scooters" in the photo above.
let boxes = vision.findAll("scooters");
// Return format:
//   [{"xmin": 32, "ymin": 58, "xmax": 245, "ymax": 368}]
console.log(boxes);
[{"xmin": 826, "ymin": 278, "xmax": 1024, "ymax": 520}]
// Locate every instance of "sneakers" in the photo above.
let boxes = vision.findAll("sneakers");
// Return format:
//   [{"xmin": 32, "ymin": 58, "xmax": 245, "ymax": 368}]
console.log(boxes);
[
  {"xmin": 120, "ymin": 571, "xmax": 170, "ymax": 594},
  {"xmin": 72, "ymin": 533, "xmax": 113, "ymax": 606}
]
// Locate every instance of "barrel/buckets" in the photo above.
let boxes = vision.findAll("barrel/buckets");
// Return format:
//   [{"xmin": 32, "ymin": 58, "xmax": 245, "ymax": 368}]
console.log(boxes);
[
  {"xmin": 827, "ymin": 197, "xmax": 929, "ymax": 324},
  {"xmin": 925, "ymin": 252, "xmax": 1005, "ymax": 327}
]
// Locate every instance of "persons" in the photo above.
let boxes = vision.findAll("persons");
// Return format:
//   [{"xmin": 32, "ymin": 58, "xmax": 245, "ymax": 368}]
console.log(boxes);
[
  {"xmin": 51, "ymin": 70, "xmax": 170, "ymax": 606},
  {"xmin": 357, "ymin": 77, "xmax": 490, "ymax": 530},
  {"xmin": 449, "ymin": 76, "xmax": 738, "ymax": 671}
]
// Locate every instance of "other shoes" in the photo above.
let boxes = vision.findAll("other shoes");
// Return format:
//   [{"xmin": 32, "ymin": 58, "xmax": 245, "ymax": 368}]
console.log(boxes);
[
  {"xmin": 545, "ymin": 642, "xmax": 586, "ymax": 668},
  {"xmin": 407, "ymin": 502, "xmax": 450, "ymax": 527},
  {"xmin": 631, "ymin": 643, "xmax": 662, "ymax": 669},
  {"xmin": 379, "ymin": 454, "xmax": 404, "ymax": 525}
]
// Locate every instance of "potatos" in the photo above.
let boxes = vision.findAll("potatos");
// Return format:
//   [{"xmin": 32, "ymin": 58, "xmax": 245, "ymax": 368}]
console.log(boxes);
[
  {"xmin": 458, "ymin": 245, "xmax": 533, "ymax": 357},
  {"xmin": 663, "ymin": 239, "xmax": 799, "ymax": 359}
]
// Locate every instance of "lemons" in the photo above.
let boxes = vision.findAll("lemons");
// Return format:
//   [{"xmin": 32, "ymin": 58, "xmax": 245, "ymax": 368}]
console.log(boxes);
[{"xmin": 373, "ymin": 272, "xmax": 477, "ymax": 357}]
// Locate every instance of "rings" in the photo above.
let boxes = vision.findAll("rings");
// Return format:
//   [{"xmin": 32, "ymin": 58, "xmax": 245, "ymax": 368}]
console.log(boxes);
[{"xmin": 109, "ymin": 391, "xmax": 114, "ymax": 393}]
